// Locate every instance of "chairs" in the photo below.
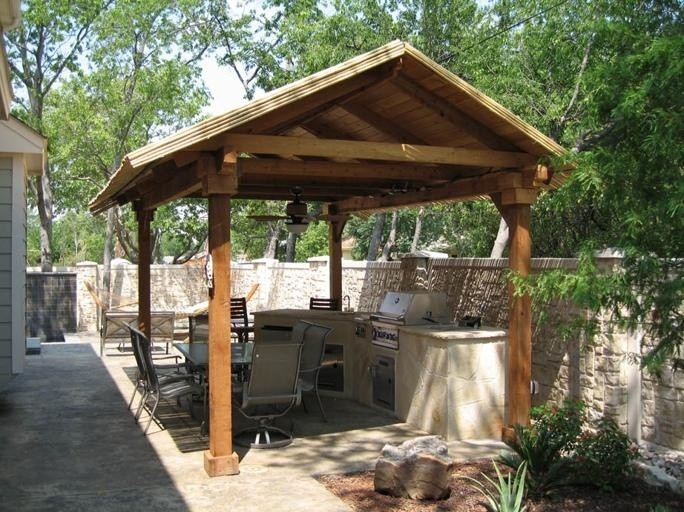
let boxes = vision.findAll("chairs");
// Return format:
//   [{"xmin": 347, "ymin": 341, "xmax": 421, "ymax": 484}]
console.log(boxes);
[{"xmin": 124, "ymin": 296, "xmax": 340, "ymax": 449}]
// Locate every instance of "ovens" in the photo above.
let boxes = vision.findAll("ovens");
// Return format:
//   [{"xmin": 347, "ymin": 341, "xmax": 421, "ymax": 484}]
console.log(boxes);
[{"xmin": 372, "ymin": 356, "xmax": 396, "ymax": 411}]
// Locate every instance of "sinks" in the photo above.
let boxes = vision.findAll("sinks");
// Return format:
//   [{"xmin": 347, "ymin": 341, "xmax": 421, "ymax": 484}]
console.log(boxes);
[{"xmin": 326, "ymin": 312, "xmax": 356, "ymax": 316}]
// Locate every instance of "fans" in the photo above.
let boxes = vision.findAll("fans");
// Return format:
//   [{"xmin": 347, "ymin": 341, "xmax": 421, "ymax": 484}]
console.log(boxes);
[{"xmin": 245, "ymin": 185, "xmax": 353, "ymax": 224}]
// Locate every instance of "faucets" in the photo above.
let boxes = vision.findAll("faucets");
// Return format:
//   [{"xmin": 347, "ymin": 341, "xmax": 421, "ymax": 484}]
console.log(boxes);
[{"xmin": 343, "ymin": 295, "xmax": 351, "ymax": 312}]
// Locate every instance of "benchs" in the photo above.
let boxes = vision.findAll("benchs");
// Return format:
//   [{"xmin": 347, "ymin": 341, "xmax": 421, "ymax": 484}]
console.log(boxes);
[{"xmin": 100, "ymin": 311, "xmax": 174, "ymax": 356}]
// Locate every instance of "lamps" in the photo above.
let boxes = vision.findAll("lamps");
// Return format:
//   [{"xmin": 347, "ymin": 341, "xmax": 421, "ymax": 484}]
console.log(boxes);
[{"xmin": 282, "ymin": 221, "xmax": 308, "ymax": 233}]
